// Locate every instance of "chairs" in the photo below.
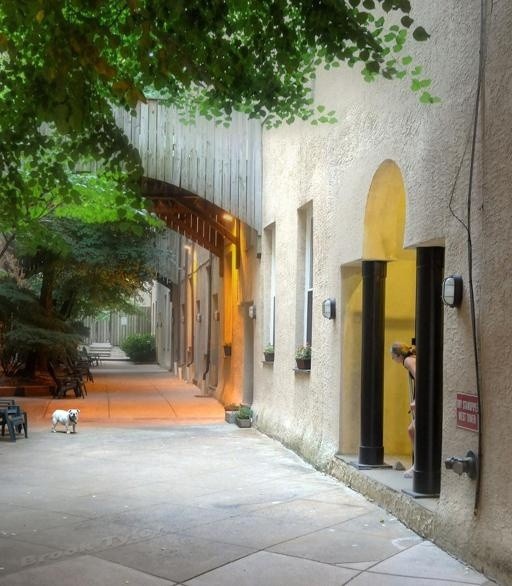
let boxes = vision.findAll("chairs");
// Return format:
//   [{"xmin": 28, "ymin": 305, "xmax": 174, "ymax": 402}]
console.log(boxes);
[
  {"xmin": 0, "ymin": 399, "xmax": 28, "ymax": 443},
  {"xmin": 47, "ymin": 346, "xmax": 99, "ymax": 399}
]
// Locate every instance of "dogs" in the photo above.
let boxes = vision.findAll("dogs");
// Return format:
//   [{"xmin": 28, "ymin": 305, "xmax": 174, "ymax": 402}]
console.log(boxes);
[{"xmin": 48, "ymin": 408, "xmax": 80, "ymax": 434}]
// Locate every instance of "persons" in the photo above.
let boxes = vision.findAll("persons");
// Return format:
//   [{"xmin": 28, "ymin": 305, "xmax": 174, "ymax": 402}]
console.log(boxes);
[{"xmin": 389, "ymin": 342, "xmax": 416, "ymax": 479}]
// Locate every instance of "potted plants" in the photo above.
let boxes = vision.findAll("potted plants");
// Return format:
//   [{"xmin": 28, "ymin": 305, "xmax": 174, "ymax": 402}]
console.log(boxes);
[
  {"xmin": 224, "ymin": 403, "xmax": 240, "ymax": 424},
  {"xmin": 263, "ymin": 346, "xmax": 274, "ymax": 361},
  {"xmin": 237, "ymin": 407, "xmax": 251, "ymax": 428}
]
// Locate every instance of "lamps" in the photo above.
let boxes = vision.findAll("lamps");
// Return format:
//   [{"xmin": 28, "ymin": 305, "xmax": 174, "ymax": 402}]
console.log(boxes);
[
  {"xmin": 294, "ymin": 345, "xmax": 311, "ymax": 369},
  {"xmin": 321, "ymin": 298, "xmax": 336, "ymax": 319},
  {"xmin": 249, "ymin": 304, "xmax": 256, "ymax": 319},
  {"xmin": 441, "ymin": 274, "xmax": 463, "ymax": 308}
]
